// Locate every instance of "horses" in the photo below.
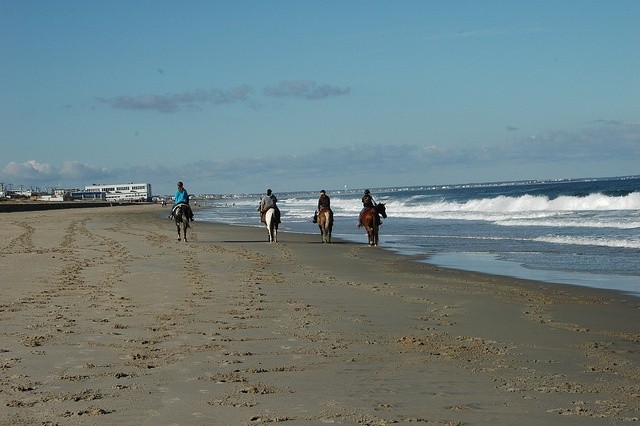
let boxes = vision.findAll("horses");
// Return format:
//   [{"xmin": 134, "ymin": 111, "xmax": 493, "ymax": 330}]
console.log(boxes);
[
  {"xmin": 360, "ymin": 203, "xmax": 387, "ymax": 246},
  {"xmin": 258, "ymin": 203, "xmax": 279, "ymax": 242},
  {"xmin": 173, "ymin": 203, "xmax": 190, "ymax": 242},
  {"xmin": 313, "ymin": 208, "xmax": 333, "ymax": 243}
]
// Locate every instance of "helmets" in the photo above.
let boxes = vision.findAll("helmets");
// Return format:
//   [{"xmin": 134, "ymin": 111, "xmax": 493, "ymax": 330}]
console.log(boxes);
[
  {"xmin": 177, "ymin": 182, "xmax": 183, "ymax": 186},
  {"xmin": 364, "ymin": 190, "xmax": 370, "ymax": 194}
]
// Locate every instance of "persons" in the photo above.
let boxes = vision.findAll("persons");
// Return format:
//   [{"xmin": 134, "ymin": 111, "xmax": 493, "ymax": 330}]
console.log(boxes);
[
  {"xmin": 167, "ymin": 181, "xmax": 194, "ymax": 222},
  {"xmin": 258, "ymin": 188, "xmax": 282, "ymax": 223},
  {"xmin": 356, "ymin": 188, "xmax": 383, "ymax": 227},
  {"xmin": 312, "ymin": 190, "xmax": 334, "ymax": 225}
]
